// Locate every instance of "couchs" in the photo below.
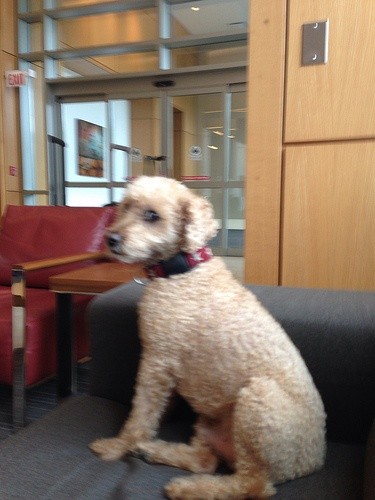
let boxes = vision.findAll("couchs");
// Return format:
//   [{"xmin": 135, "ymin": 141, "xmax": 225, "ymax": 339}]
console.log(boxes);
[{"xmin": 0, "ymin": 279, "xmax": 375, "ymax": 499}]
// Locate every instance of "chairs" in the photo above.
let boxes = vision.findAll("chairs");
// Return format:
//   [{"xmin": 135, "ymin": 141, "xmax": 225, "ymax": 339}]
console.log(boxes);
[{"xmin": 0, "ymin": 204, "xmax": 119, "ymax": 433}]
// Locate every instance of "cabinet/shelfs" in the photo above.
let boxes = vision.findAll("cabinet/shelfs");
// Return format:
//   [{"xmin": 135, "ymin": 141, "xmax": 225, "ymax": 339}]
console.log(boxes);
[{"xmin": 244, "ymin": 0, "xmax": 374, "ymax": 293}]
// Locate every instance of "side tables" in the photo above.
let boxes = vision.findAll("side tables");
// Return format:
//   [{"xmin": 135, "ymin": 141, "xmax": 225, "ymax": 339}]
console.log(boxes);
[{"xmin": 50, "ymin": 261, "xmax": 150, "ymax": 399}]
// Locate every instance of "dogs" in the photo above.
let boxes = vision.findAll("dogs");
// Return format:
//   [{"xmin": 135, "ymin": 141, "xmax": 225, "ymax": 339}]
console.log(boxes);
[{"xmin": 87, "ymin": 175, "xmax": 326, "ymax": 500}]
